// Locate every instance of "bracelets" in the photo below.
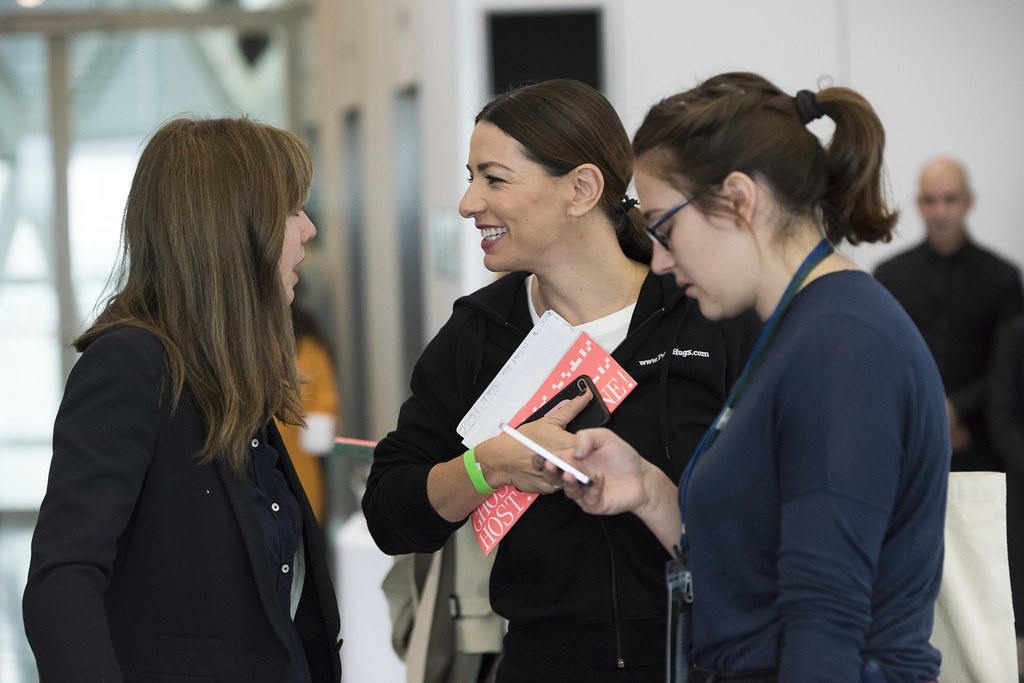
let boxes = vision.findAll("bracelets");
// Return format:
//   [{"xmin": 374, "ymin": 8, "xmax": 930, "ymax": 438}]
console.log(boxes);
[{"xmin": 463, "ymin": 447, "xmax": 499, "ymax": 494}]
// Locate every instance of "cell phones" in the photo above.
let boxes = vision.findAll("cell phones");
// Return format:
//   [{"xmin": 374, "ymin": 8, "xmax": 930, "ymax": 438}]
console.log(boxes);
[
  {"xmin": 514, "ymin": 375, "xmax": 610, "ymax": 434},
  {"xmin": 499, "ymin": 423, "xmax": 590, "ymax": 485}
]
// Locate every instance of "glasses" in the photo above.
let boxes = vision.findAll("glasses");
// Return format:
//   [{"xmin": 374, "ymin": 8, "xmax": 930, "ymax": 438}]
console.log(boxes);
[{"xmin": 646, "ymin": 185, "xmax": 712, "ymax": 252}]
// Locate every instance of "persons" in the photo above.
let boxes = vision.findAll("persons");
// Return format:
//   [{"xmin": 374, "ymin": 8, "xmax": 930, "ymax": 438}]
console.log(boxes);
[
  {"xmin": 359, "ymin": 77, "xmax": 763, "ymax": 683},
  {"xmin": 872, "ymin": 156, "xmax": 1024, "ymax": 683},
  {"xmin": 21, "ymin": 116, "xmax": 344, "ymax": 683},
  {"xmin": 531, "ymin": 71, "xmax": 953, "ymax": 683},
  {"xmin": 271, "ymin": 304, "xmax": 340, "ymax": 527}
]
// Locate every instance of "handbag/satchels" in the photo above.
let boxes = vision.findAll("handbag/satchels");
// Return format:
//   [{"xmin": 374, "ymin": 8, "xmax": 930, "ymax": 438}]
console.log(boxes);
[{"xmin": 381, "ymin": 512, "xmax": 509, "ymax": 683}]
